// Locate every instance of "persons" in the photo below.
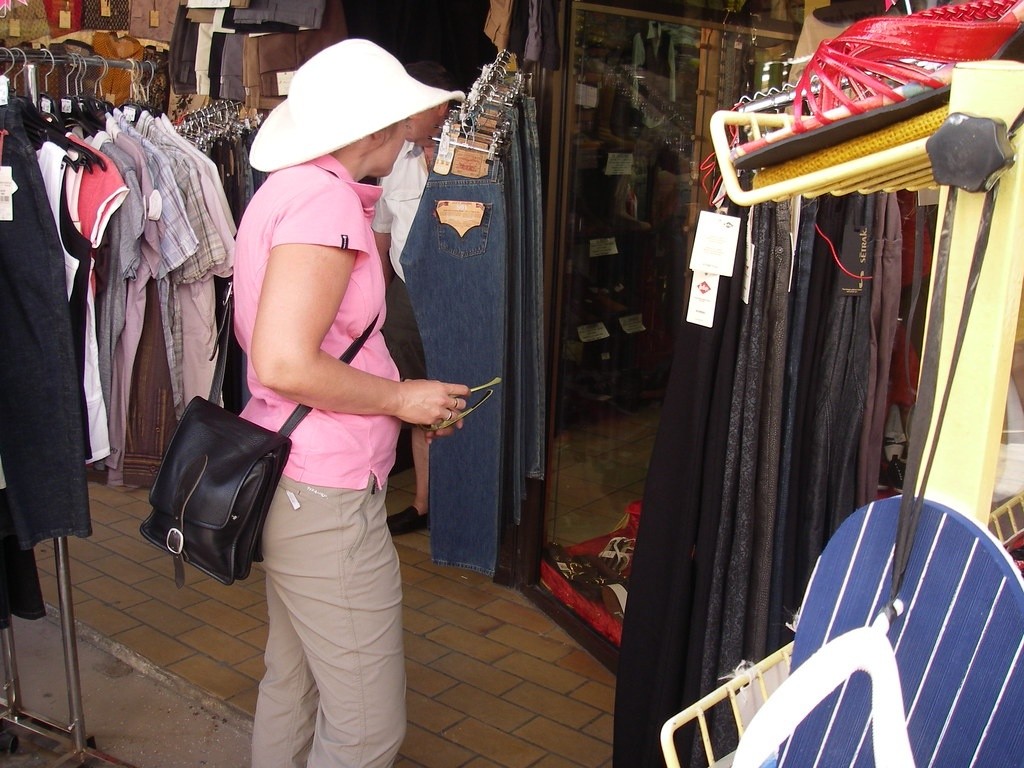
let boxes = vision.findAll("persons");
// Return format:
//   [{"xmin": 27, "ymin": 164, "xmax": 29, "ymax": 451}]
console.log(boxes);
[
  {"xmin": 371, "ymin": 99, "xmax": 451, "ymax": 533},
  {"xmin": 232, "ymin": 38, "xmax": 468, "ymax": 768}
]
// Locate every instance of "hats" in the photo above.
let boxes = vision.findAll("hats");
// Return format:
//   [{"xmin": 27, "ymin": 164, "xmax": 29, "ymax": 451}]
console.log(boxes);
[
  {"xmin": 404, "ymin": 57, "xmax": 454, "ymax": 91},
  {"xmin": 246, "ymin": 37, "xmax": 467, "ymax": 172}
]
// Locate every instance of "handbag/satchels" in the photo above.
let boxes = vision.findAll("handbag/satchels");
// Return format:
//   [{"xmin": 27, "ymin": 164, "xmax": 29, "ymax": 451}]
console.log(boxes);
[{"xmin": 137, "ymin": 395, "xmax": 292, "ymax": 591}]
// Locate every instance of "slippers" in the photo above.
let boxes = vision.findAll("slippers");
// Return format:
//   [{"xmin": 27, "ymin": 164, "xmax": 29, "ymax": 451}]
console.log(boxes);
[{"xmin": 728, "ymin": 492, "xmax": 1024, "ymax": 768}]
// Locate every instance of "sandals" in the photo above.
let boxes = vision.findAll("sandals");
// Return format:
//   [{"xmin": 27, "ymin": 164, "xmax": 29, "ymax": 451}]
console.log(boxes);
[{"xmin": 731, "ymin": 0, "xmax": 1024, "ymax": 171}]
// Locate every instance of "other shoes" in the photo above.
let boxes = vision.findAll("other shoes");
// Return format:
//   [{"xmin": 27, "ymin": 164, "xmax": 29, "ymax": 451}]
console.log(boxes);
[{"xmin": 386, "ymin": 505, "xmax": 428, "ymax": 536}]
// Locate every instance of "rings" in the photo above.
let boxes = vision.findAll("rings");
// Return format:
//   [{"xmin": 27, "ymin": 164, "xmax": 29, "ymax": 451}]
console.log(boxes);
[
  {"xmin": 444, "ymin": 408, "xmax": 452, "ymax": 422},
  {"xmin": 452, "ymin": 396, "xmax": 459, "ymax": 408}
]
